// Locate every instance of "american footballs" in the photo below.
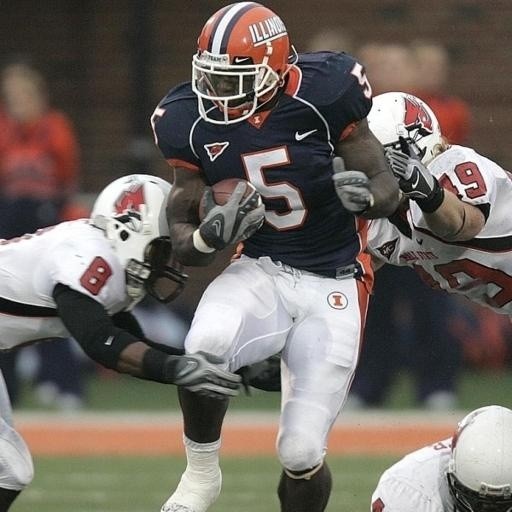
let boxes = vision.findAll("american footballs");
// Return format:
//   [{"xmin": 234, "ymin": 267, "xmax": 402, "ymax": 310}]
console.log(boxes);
[{"xmin": 199, "ymin": 178, "xmax": 262, "ymax": 222}]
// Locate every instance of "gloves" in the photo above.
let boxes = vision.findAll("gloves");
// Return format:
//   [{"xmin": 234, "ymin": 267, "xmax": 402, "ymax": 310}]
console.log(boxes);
[
  {"xmin": 331, "ymin": 156, "xmax": 376, "ymax": 214},
  {"xmin": 192, "ymin": 181, "xmax": 267, "ymax": 255},
  {"xmin": 382, "ymin": 136, "xmax": 444, "ymax": 214},
  {"xmin": 236, "ymin": 354, "xmax": 282, "ymax": 393},
  {"xmin": 141, "ymin": 346, "xmax": 243, "ymax": 402}
]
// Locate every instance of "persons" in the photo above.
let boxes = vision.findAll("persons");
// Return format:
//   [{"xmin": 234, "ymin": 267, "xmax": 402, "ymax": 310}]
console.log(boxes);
[
  {"xmin": 147, "ymin": 2, "xmax": 402, "ymax": 512},
  {"xmin": 307, "ymin": 30, "xmax": 471, "ymax": 405},
  {"xmin": 0, "ymin": 171, "xmax": 281, "ymax": 511},
  {"xmin": 368, "ymin": 403, "xmax": 512, "ymax": 511},
  {"xmin": 1, "ymin": 63, "xmax": 86, "ymax": 404},
  {"xmin": 360, "ymin": 90, "xmax": 512, "ymax": 324}
]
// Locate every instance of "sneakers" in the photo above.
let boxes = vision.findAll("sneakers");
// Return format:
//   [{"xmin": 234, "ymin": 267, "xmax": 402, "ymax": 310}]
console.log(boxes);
[{"xmin": 158, "ymin": 467, "xmax": 224, "ymax": 512}]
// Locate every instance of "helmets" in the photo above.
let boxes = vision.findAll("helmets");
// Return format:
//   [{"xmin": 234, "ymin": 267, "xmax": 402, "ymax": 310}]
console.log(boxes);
[
  {"xmin": 366, "ymin": 92, "xmax": 448, "ymax": 169},
  {"xmin": 445, "ymin": 405, "xmax": 512, "ymax": 512},
  {"xmin": 190, "ymin": 1, "xmax": 298, "ymax": 125},
  {"xmin": 87, "ymin": 173, "xmax": 188, "ymax": 304}
]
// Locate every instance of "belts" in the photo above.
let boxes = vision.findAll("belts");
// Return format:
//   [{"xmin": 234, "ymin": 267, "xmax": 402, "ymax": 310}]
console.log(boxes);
[{"xmin": 316, "ymin": 262, "xmax": 364, "ymax": 280}]
